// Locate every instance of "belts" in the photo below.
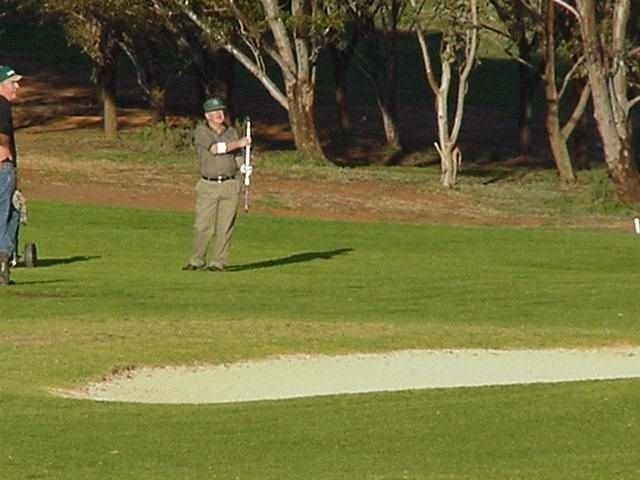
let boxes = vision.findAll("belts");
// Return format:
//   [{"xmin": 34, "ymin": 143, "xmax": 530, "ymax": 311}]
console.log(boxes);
[{"xmin": 201, "ymin": 174, "xmax": 237, "ymax": 182}]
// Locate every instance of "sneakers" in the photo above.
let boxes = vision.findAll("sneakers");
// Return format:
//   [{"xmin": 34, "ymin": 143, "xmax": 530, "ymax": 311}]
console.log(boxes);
[{"xmin": 181, "ymin": 259, "xmax": 224, "ymax": 272}]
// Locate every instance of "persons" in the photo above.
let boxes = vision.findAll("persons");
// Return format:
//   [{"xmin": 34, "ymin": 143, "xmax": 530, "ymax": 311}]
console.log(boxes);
[
  {"xmin": 182, "ymin": 98, "xmax": 252, "ymax": 271},
  {"xmin": 0, "ymin": 65, "xmax": 23, "ymax": 268}
]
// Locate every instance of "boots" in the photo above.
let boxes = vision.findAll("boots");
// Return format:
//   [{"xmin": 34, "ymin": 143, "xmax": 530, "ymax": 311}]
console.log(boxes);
[{"xmin": 0, "ymin": 253, "xmax": 14, "ymax": 285}]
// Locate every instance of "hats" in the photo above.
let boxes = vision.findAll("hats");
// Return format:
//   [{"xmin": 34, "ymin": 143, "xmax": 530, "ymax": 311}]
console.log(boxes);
[
  {"xmin": 0, "ymin": 65, "xmax": 23, "ymax": 83},
  {"xmin": 202, "ymin": 96, "xmax": 228, "ymax": 111}
]
